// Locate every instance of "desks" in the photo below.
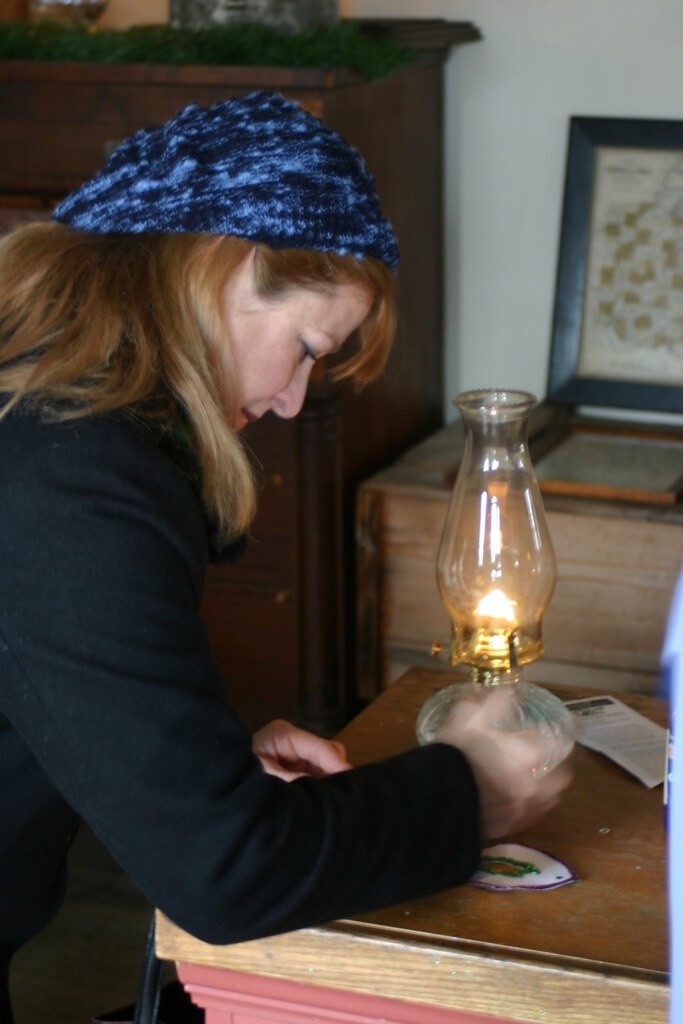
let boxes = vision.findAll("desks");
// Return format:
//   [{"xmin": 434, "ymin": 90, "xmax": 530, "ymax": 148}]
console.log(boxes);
[{"xmin": 152, "ymin": 664, "xmax": 680, "ymax": 1024}]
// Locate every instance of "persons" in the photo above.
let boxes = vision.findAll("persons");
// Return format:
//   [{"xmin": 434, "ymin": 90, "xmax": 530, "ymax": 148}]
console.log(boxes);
[{"xmin": 0, "ymin": 91, "xmax": 574, "ymax": 1024}]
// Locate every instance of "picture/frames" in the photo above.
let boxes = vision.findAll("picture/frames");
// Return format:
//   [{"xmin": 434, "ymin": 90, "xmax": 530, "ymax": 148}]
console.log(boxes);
[
  {"xmin": 446, "ymin": 411, "xmax": 683, "ymax": 509},
  {"xmin": 549, "ymin": 118, "xmax": 683, "ymax": 416}
]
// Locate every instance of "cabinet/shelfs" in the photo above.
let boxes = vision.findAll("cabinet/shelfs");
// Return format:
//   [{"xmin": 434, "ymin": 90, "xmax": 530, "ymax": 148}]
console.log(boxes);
[{"xmin": 0, "ymin": 19, "xmax": 682, "ymax": 737}]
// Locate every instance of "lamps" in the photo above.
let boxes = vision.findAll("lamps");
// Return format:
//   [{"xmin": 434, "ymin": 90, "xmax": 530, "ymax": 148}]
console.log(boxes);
[{"xmin": 414, "ymin": 388, "xmax": 558, "ymax": 746}]
